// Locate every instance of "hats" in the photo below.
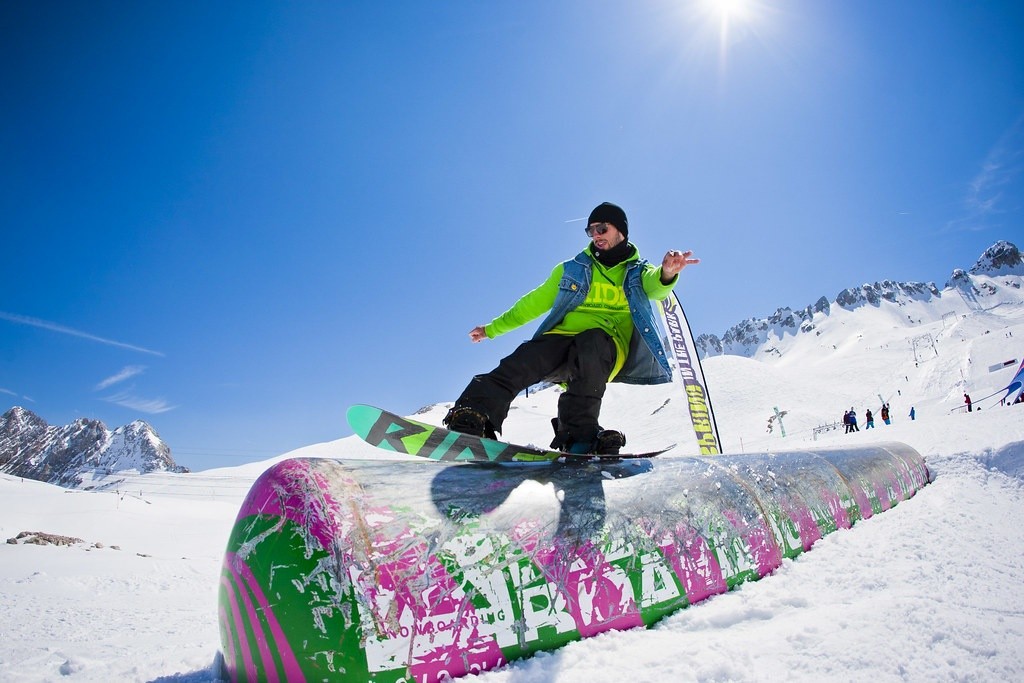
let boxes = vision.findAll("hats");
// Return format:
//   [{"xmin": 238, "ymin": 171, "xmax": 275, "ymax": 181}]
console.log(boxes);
[{"xmin": 588, "ymin": 202, "xmax": 628, "ymax": 239}]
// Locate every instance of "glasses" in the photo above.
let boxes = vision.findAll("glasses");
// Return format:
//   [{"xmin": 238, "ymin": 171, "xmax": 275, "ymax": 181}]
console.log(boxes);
[{"xmin": 585, "ymin": 223, "xmax": 610, "ymax": 237}]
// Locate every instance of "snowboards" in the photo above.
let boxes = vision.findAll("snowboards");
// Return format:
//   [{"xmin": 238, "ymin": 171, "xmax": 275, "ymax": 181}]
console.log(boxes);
[
  {"xmin": 345, "ymin": 402, "xmax": 679, "ymax": 464},
  {"xmin": 656, "ymin": 288, "xmax": 723, "ymax": 456}
]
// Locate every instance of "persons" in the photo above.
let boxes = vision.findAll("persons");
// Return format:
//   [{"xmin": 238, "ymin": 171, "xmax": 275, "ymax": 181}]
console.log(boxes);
[
  {"xmin": 444, "ymin": 202, "xmax": 700, "ymax": 455},
  {"xmin": 844, "ymin": 407, "xmax": 859, "ymax": 434},
  {"xmin": 964, "ymin": 394, "xmax": 972, "ymax": 412},
  {"xmin": 866, "ymin": 409, "xmax": 874, "ymax": 429},
  {"xmin": 910, "ymin": 407, "xmax": 915, "ymax": 420},
  {"xmin": 882, "ymin": 404, "xmax": 890, "ymax": 425}
]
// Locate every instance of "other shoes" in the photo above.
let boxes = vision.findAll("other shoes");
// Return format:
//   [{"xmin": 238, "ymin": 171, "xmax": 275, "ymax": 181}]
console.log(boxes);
[
  {"xmin": 448, "ymin": 409, "xmax": 487, "ymax": 436},
  {"xmin": 560, "ymin": 426, "xmax": 623, "ymax": 454}
]
